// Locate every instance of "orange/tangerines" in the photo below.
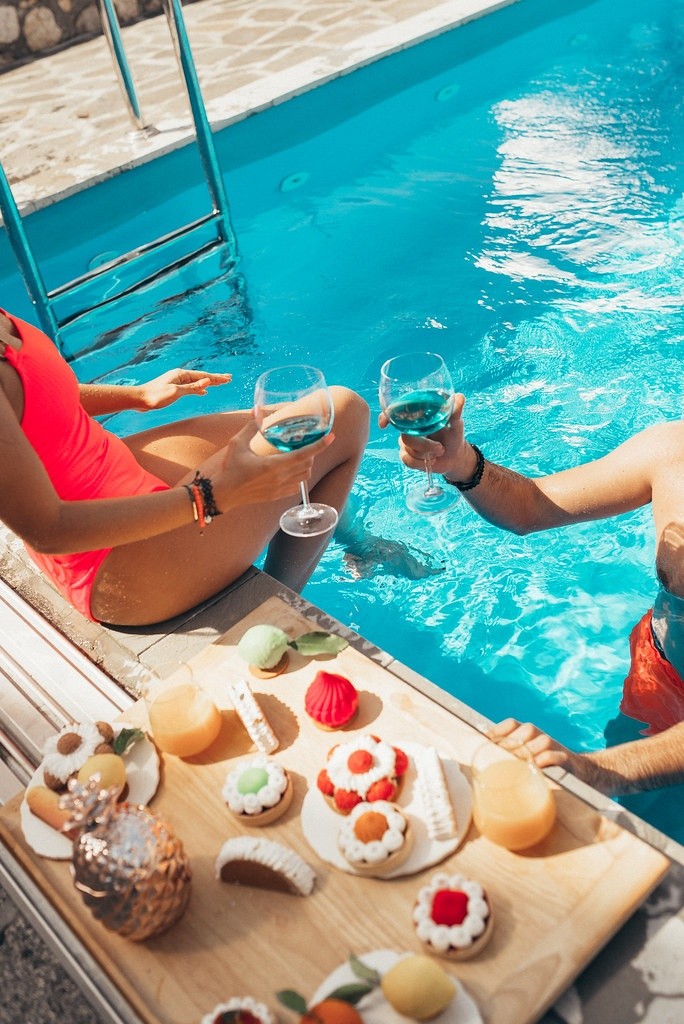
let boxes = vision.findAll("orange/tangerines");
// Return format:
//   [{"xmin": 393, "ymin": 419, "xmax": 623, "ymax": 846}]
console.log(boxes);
[{"xmin": 300, "ymin": 998, "xmax": 362, "ymax": 1024}]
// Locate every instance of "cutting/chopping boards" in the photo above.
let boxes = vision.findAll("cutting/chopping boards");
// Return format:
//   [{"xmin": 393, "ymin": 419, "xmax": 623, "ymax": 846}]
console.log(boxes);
[{"xmin": 0, "ymin": 595, "xmax": 673, "ymax": 1024}]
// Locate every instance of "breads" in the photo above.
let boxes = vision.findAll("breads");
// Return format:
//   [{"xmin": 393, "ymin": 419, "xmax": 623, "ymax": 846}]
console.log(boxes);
[{"xmin": 26, "ymin": 786, "xmax": 79, "ymax": 840}]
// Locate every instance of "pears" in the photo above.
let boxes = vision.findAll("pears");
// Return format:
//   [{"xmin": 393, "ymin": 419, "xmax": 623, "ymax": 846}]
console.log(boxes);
[
  {"xmin": 378, "ymin": 952, "xmax": 456, "ymax": 1021},
  {"xmin": 77, "ymin": 753, "xmax": 126, "ymax": 800}
]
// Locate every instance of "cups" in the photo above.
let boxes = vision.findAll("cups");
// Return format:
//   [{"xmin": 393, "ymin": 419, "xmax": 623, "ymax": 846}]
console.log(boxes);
[
  {"xmin": 470, "ymin": 738, "xmax": 556, "ymax": 851},
  {"xmin": 139, "ymin": 663, "xmax": 223, "ymax": 761}
]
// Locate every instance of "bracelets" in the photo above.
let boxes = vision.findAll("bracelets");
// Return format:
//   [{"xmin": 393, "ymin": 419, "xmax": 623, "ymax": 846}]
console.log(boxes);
[
  {"xmin": 442, "ymin": 444, "xmax": 485, "ymax": 492},
  {"xmin": 182, "ymin": 470, "xmax": 224, "ymax": 536}
]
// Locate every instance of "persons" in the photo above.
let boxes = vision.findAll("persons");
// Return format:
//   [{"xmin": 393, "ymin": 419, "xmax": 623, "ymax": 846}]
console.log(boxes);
[
  {"xmin": 379, "ymin": 394, "xmax": 684, "ymax": 799},
  {"xmin": 0, "ymin": 310, "xmax": 370, "ymax": 624}
]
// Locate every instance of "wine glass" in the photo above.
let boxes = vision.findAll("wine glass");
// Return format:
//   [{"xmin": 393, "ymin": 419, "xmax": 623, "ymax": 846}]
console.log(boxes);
[
  {"xmin": 252, "ymin": 366, "xmax": 339, "ymax": 536},
  {"xmin": 377, "ymin": 353, "xmax": 461, "ymax": 516}
]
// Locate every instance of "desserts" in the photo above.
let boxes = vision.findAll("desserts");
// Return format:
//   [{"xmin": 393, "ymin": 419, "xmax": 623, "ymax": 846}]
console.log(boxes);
[{"xmin": 42, "ymin": 625, "xmax": 491, "ymax": 1024}]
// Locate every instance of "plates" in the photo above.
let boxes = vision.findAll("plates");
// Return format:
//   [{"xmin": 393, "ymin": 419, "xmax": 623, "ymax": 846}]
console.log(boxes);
[
  {"xmin": 300, "ymin": 750, "xmax": 472, "ymax": 878},
  {"xmin": 307, "ymin": 948, "xmax": 484, "ymax": 1024},
  {"xmin": 19, "ymin": 723, "xmax": 159, "ymax": 860}
]
